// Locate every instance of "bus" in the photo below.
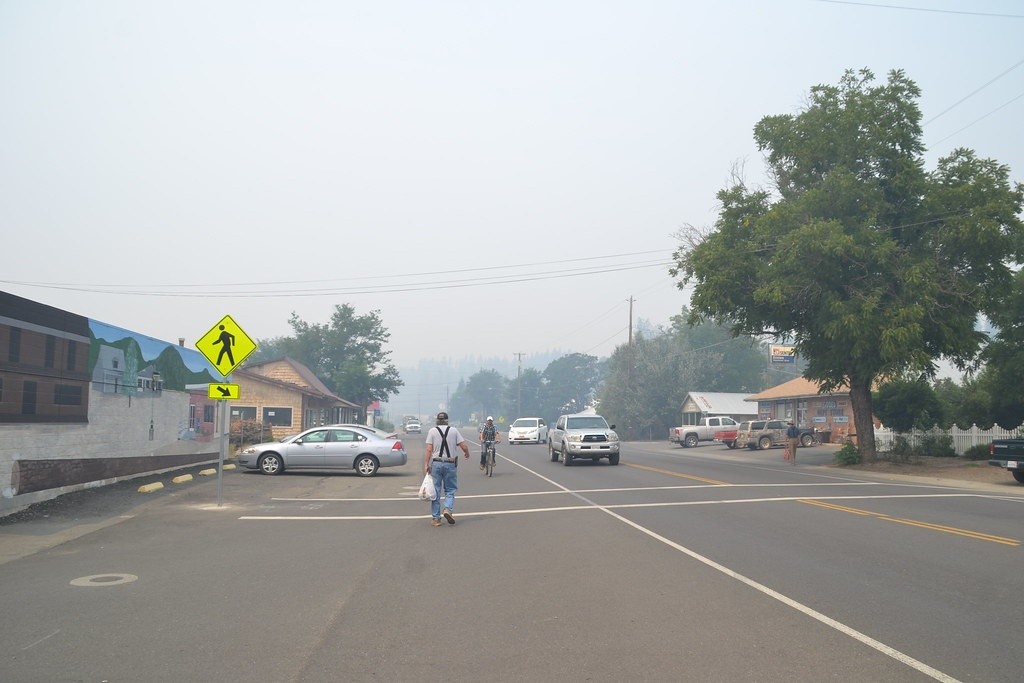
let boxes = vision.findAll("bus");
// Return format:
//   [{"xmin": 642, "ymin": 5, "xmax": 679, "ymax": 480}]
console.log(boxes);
[{"xmin": 402, "ymin": 415, "xmax": 418, "ymax": 431}]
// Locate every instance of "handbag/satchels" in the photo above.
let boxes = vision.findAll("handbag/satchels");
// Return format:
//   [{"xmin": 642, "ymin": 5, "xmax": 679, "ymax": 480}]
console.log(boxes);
[{"xmin": 418, "ymin": 472, "xmax": 437, "ymax": 501}]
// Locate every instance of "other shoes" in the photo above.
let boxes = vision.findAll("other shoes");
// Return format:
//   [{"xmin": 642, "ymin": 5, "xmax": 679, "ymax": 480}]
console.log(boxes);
[
  {"xmin": 431, "ymin": 519, "xmax": 441, "ymax": 526},
  {"xmin": 480, "ymin": 464, "xmax": 484, "ymax": 470},
  {"xmin": 443, "ymin": 509, "xmax": 455, "ymax": 524},
  {"xmin": 490, "ymin": 462, "xmax": 496, "ymax": 466}
]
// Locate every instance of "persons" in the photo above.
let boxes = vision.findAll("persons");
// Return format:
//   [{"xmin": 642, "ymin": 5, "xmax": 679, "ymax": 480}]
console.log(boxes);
[
  {"xmin": 784, "ymin": 419, "xmax": 801, "ymax": 465},
  {"xmin": 425, "ymin": 412, "xmax": 470, "ymax": 526},
  {"xmin": 480, "ymin": 416, "xmax": 501, "ymax": 470}
]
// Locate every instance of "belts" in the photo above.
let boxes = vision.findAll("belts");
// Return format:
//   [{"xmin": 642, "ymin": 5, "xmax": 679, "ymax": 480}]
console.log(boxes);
[{"xmin": 433, "ymin": 459, "xmax": 454, "ymax": 463}]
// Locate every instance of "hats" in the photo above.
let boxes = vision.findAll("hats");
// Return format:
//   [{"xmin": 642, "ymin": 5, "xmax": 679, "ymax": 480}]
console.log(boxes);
[
  {"xmin": 437, "ymin": 412, "xmax": 448, "ymax": 419},
  {"xmin": 487, "ymin": 416, "xmax": 493, "ymax": 421}
]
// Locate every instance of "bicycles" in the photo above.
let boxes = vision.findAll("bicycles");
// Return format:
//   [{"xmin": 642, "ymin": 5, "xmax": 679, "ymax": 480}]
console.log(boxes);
[
  {"xmin": 479, "ymin": 440, "xmax": 498, "ymax": 477},
  {"xmin": 809, "ymin": 425, "xmax": 822, "ymax": 445}
]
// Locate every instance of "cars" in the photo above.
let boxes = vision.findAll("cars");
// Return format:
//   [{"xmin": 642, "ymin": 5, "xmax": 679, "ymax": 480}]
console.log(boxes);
[
  {"xmin": 281, "ymin": 423, "xmax": 398, "ymax": 442},
  {"xmin": 238, "ymin": 426, "xmax": 407, "ymax": 477},
  {"xmin": 405, "ymin": 420, "xmax": 423, "ymax": 434},
  {"xmin": 508, "ymin": 418, "xmax": 548, "ymax": 444}
]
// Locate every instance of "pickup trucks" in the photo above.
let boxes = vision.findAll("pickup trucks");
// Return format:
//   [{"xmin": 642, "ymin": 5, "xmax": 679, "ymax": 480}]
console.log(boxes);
[
  {"xmin": 669, "ymin": 416, "xmax": 740, "ymax": 448},
  {"xmin": 988, "ymin": 438, "xmax": 1024, "ymax": 484},
  {"xmin": 713, "ymin": 431, "xmax": 737, "ymax": 449}
]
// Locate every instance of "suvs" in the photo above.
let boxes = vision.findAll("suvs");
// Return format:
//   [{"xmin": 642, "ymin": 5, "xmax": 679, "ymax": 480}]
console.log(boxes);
[
  {"xmin": 548, "ymin": 415, "xmax": 620, "ymax": 467},
  {"xmin": 736, "ymin": 417, "xmax": 813, "ymax": 450}
]
